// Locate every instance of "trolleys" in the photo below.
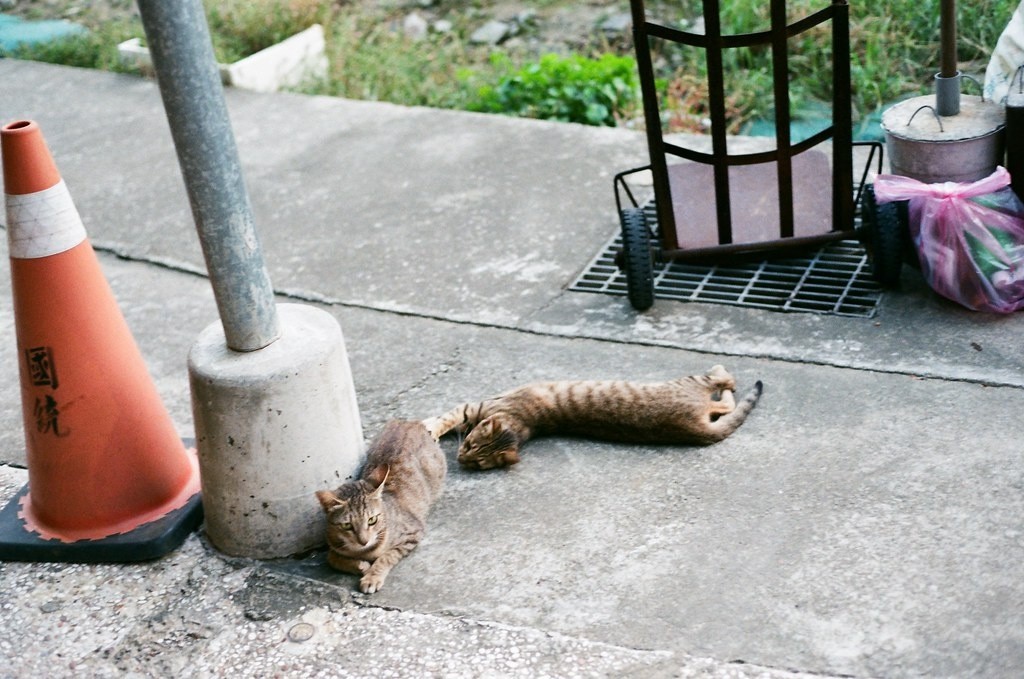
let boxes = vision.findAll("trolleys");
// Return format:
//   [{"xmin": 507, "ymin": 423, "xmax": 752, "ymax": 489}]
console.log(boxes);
[{"xmin": 611, "ymin": 0, "xmax": 911, "ymax": 314}]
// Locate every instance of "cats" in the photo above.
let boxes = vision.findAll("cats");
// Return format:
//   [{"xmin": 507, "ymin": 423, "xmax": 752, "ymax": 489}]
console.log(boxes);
[
  {"xmin": 454, "ymin": 365, "xmax": 763, "ymax": 471},
  {"xmin": 312, "ymin": 400, "xmax": 483, "ymax": 594}
]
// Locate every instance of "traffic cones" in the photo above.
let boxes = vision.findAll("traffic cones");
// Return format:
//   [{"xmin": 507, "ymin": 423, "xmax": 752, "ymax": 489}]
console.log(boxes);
[{"xmin": 0, "ymin": 119, "xmax": 204, "ymax": 566}]
